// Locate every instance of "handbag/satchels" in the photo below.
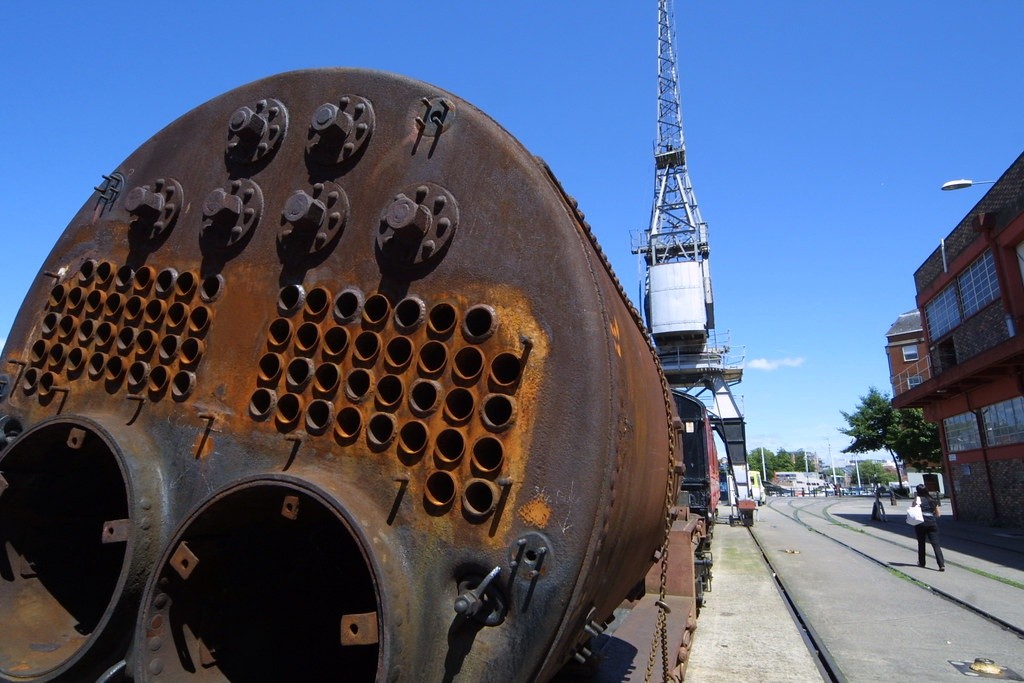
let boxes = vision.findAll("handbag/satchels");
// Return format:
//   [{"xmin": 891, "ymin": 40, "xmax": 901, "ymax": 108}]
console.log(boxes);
[{"xmin": 906, "ymin": 496, "xmax": 924, "ymax": 526}]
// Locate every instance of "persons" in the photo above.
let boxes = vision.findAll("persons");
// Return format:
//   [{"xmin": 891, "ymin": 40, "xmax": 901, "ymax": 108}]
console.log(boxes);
[{"xmin": 911, "ymin": 484, "xmax": 946, "ymax": 571}]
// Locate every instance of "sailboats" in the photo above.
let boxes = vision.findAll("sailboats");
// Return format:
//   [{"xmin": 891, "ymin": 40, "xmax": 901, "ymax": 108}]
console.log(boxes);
[{"xmin": 810, "ymin": 444, "xmax": 846, "ymax": 496}]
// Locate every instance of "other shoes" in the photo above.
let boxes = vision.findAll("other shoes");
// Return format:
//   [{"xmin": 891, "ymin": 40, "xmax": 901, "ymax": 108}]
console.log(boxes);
[
  {"xmin": 917, "ymin": 561, "xmax": 925, "ymax": 567},
  {"xmin": 939, "ymin": 567, "xmax": 945, "ymax": 571}
]
